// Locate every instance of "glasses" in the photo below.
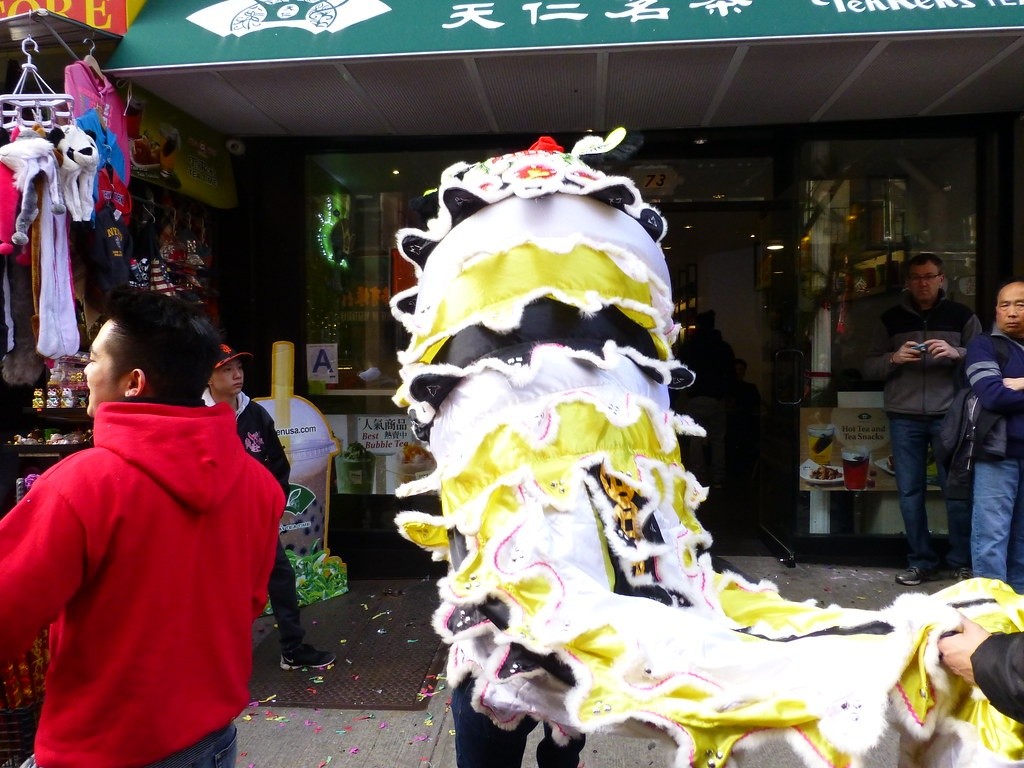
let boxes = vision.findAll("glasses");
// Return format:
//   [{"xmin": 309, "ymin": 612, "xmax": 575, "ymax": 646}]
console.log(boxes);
[{"xmin": 907, "ymin": 272, "xmax": 940, "ymax": 285}]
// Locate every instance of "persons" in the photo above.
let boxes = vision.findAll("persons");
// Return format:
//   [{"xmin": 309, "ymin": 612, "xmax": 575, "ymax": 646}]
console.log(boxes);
[
  {"xmin": 0, "ymin": 290, "xmax": 286, "ymax": 768},
  {"xmin": 938, "ymin": 609, "xmax": 1024, "ymax": 724},
  {"xmin": 451, "ymin": 671, "xmax": 586, "ymax": 768},
  {"xmin": 862, "ymin": 252, "xmax": 983, "ymax": 584},
  {"xmin": 676, "ymin": 309, "xmax": 761, "ymax": 505},
  {"xmin": 964, "ymin": 281, "xmax": 1024, "ymax": 595},
  {"xmin": 202, "ymin": 343, "xmax": 337, "ymax": 670}
]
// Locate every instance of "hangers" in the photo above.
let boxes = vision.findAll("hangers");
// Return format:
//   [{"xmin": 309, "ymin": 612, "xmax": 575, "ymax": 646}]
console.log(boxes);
[
  {"xmin": 140, "ymin": 200, "xmax": 207, "ymax": 234},
  {"xmin": 81, "ymin": 39, "xmax": 106, "ymax": 81}
]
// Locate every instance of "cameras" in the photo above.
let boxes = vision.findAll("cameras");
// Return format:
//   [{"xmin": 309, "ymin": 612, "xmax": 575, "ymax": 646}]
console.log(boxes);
[{"xmin": 910, "ymin": 344, "xmax": 928, "ymax": 353}]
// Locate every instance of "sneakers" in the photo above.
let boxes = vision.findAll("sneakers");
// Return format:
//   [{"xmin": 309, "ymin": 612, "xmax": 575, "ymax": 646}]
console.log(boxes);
[
  {"xmin": 896, "ymin": 561, "xmax": 941, "ymax": 585},
  {"xmin": 948, "ymin": 565, "xmax": 972, "ymax": 583},
  {"xmin": 279, "ymin": 644, "xmax": 338, "ymax": 669}
]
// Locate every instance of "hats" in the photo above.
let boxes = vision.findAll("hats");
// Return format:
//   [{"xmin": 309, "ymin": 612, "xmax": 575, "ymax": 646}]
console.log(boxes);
[{"xmin": 212, "ymin": 344, "xmax": 254, "ymax": 368}]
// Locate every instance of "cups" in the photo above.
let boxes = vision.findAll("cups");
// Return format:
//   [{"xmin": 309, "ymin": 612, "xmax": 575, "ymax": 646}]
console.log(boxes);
[
  {"xmin": 332, "ymin": 436, "xmax": 376, "ymax": 495},
  {"xmin": 841, "ymin": 445, "xmax": 870, "ymax": 491},
  {"xmin": 252, "ymin": 342, "xmax": 336, "ymax": 556},
  {"xmin": 397, "ymin": 455, "xmax": 436, "ymax": 487},
  {"xmin": 807, "ymin": 423, "xmax": 836, "ymax": 465}
]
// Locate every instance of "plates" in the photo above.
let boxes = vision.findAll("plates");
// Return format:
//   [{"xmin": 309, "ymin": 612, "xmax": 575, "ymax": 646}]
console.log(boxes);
[
  {"xmin": 874, "ymin": 455, "xmax": 895, "ymax": 476},
  {"xmin": 801, "ymin": 458, "xmax": 844, "ymax": 485}
]
